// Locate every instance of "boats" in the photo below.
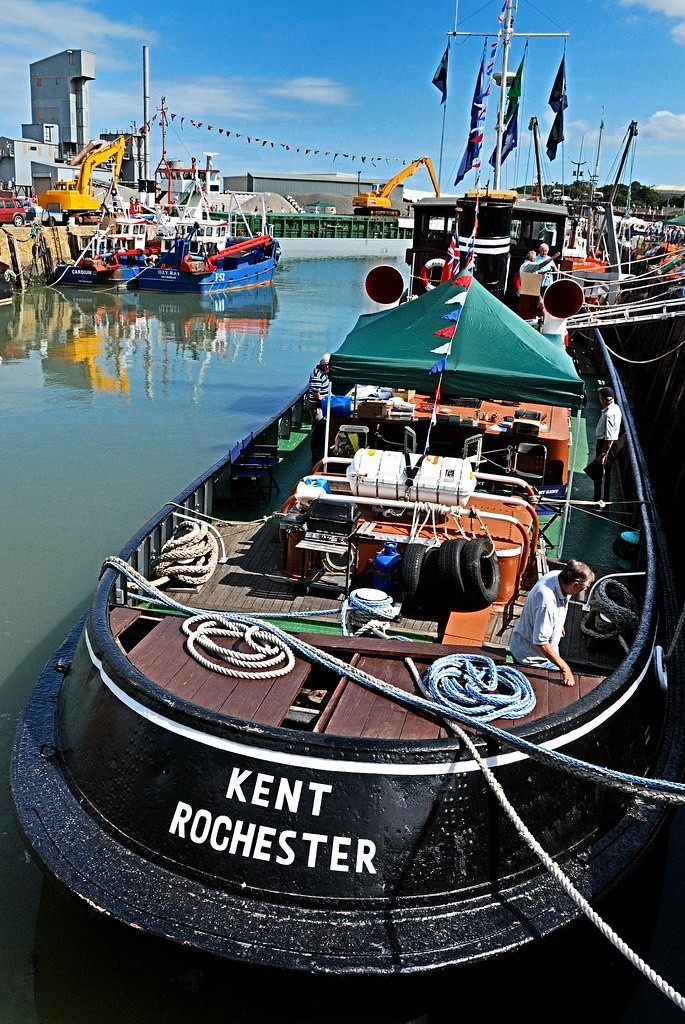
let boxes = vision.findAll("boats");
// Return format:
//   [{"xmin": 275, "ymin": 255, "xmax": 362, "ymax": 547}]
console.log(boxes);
[
  {"xmin": 6, "ymin": 0, "xmax": 685, "ymax": 1024},
  {"xmin": 53, "ymin": 167, "xmax": 282, "ymax": 293}
]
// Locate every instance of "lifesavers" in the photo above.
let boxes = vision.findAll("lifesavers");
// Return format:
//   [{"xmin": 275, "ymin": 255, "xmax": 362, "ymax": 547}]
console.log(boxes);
[
  {"xmin": 419, "ymin": 257, "xmax": 452, "ymax": 292},
  {"xmin": 397, "ymin": 537, "xmax": 503, "ymax": 614}
]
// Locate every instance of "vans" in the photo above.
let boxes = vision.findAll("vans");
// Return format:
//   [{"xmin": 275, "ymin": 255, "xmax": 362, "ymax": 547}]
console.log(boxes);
[{"xmin": 0, "ymin": 197, "xmax": 66, "ymax": 228}]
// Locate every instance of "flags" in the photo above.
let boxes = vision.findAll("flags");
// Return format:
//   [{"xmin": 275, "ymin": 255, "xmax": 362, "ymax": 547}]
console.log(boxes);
[
  {"xmin": 454, "ymin": 57, "xmax": 484, "ymax": 186},
  {"xmin": 488, "ymin": 54, "xmax": 525, "ymax": 172},
  {"xmin": 432, "ymin": 47, "xmax": 449, "ymax": 104},
  {"xmin": 546, "ymin": 52, "xmax": 568, "ymax": 160}
]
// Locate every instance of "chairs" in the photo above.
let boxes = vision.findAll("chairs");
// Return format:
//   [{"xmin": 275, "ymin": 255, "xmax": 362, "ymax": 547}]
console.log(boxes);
[
  {"xmin": 513, "ymin": 442, "xmax": 548, "ymax": 486},
  {"xmin": 298, "ymin": 499, "xmax": 352, "ymax": 600},
  {"xmin": 534, "ymin": 483, "xmax": 569, "ymax": 549},
  {"xmin": 228, "ymin": 432, "xmax": 281, "ymax": 505}
]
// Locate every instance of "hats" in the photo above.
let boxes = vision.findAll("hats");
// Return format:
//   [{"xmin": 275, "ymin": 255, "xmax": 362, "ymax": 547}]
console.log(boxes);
[{"xmin": 598, "ymin": 387, "xmax": 614, "ymax": 400}]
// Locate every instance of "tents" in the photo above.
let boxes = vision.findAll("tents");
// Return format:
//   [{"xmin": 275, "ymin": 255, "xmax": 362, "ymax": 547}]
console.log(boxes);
[
  {"xmin": 306, "ymin": 201, "xmax": 335, "ymax": 214},
  {"xmin": 322, "ymin": 268, "xmax": 585, "ymax": 559}
]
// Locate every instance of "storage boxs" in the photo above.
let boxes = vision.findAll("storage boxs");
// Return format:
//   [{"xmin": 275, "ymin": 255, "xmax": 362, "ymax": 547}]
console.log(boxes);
[
  {"xmin": 321, "ymin": 396, "xmax": 352, "ymax": 420},
  {"xmin": 357, "ymin": 402, "xmax": 388, "ymax": 419},
  {"xmin": 513, "ymin": 418, "xmax": 540, "ymax": 437}
]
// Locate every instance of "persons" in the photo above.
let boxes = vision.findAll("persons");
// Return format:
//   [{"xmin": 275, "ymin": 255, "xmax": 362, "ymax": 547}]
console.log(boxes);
[
  {"xmin": 23, "ymin": 197, "xmax": 32, "ymax": 207},
  {"xmin": 630, "ymin": 224, "xmax": 685, "ymax": 247},
  {"xmin": 518, "ymin": 243, "xmax": 560, "ymax": 323},
  {"xmin": 281, "ymin": 207, "xmax": 294, "ymax": 213},
  {"xmin": 510, "ymin": 559, "xmax": 595, "ymax": 686},
  {"xmin": 330, "ymin": 208, "xmax": 334, "ymax": 214},
  {"xmin": 267, "ymin": 206, "xmax": 273, "ymax": 213},
  {"xmin": 587, "ymin": 387, "xmax": 622, "ymax": 514},
  {"xmin": 210, "ymin": 203, "xmax": 225, "ymax": 211},
  {"xmin": 254, "ymin": 205, "xmax": 258, "ymax": 212},
  {"xmin": 309, "ymin": 353, "xmax": 331, "ymax": 447}
]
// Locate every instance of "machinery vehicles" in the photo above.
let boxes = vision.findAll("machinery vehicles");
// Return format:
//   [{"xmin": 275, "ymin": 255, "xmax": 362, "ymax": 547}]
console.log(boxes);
[
  {"xmin": 38, "ymin": 134, "xmax": 126, "ymax": 227},
  {"xmin": 351, "ymin": 154, "xmax": 440, "ymax": 217}
]
